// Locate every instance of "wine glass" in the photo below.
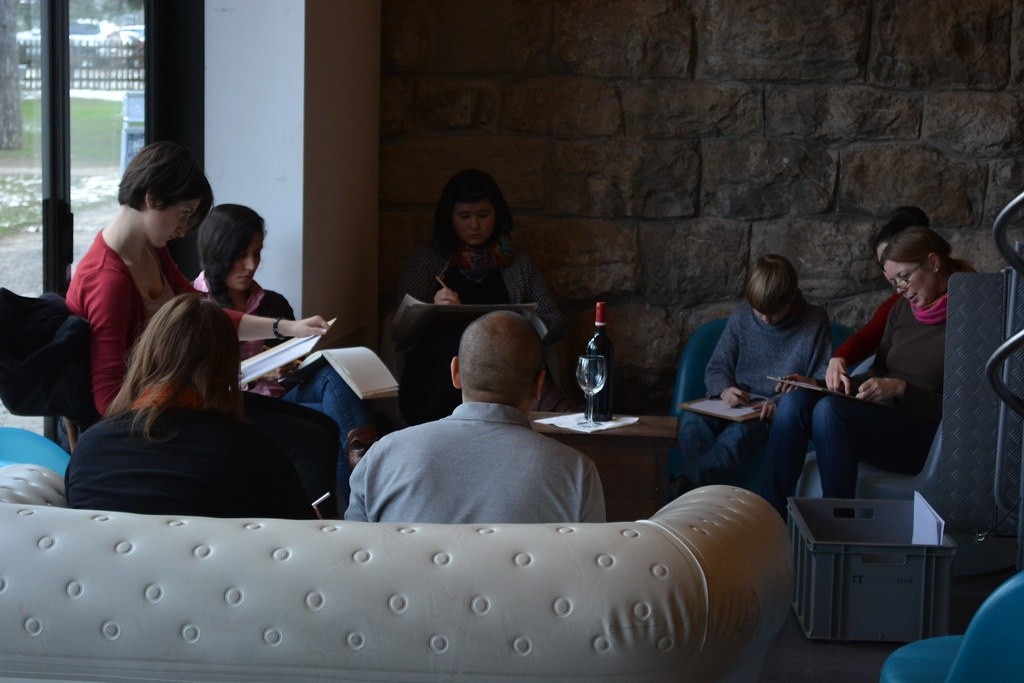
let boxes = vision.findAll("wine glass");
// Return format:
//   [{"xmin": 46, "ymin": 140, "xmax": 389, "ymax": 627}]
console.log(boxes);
[{"xmin": 575, "ymin": 354, "xmax": 608, "ymax": 429}]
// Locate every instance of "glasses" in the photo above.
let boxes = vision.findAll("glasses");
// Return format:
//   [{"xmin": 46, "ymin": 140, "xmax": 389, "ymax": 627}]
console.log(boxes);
[{"xmin": 893, "ymin": 260, "xmax": 923, "ymax": 289}]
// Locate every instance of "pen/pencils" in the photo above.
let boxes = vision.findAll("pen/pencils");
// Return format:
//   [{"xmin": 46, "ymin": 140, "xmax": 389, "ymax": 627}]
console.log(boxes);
[
  {"xmin": 436, "ymin": 275, "xmax": 447, "ymax": 288},
  {"xmin": 262, "ymin": 345, "xmax": 303, "ymax": 365},
  {"xmin": 726, "ymin": 375, "xmax": 752, "ymax": 407},
  {"xmin": 843, "ymin": 372, "xmax": 861, "ymax": 386}
]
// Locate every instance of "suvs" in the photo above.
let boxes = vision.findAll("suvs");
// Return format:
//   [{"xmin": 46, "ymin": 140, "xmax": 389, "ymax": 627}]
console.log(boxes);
[{"xmin": 17, "ymin": 17, "xmax": 118, "ymax": 67}]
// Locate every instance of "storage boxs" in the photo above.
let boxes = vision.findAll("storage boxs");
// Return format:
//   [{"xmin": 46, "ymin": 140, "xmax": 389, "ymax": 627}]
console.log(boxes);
[{"xmin": 786, "ymin": 495, "xmax": 956, "ymax": 646}]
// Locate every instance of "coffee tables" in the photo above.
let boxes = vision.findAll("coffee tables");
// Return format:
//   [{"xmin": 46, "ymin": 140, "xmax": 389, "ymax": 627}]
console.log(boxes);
[{"xmin": 520, "ymin": 411, "xmax": 682, "ymax": 520}]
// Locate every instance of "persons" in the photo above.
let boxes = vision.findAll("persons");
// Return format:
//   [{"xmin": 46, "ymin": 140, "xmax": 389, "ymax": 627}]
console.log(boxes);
[
  {"xmin": 189, "ymin": 203, "xmax": 382, "ymax": 521},
  {"xmin": 401, "ymin": 168, "xmax": 572, "ymax": 426},
  {"xmin": 824, "ymin": 205, "xmax": 976, "ymax": 397},
  {"xmin": 66, "ymin": 142, "xmax": 328, "ymax": 414},
  {"xmin": 677, "ymin": 254, "xmax": 836, "ymax": 488},
  {"xmin": 761, "ymin": 226, "xmax": 976, "ymax": 519},
  {"xmin": 342, "ymin": 309, "xmax": 606, "ymax": 523},
  {"xmin": 65, "ymin": 292, "xmax": 339, "ymax": 519}
]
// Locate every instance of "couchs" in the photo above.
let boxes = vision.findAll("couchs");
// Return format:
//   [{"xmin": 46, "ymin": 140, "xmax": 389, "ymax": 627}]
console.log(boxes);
[{"xmin": 0, "ymin": 461, "xmax": 796, "ymax": 683}]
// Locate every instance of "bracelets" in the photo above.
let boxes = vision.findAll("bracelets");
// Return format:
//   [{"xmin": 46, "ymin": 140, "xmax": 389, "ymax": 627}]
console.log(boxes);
[{"xmin": 273, "ymin": 317, "xmax": 286, "ymax": 341}]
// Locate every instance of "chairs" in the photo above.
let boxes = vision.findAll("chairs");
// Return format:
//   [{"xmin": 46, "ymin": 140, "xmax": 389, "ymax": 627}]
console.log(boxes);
[
  {"xmin": 0, "ymin": 427, "xmax": 71, "ymax": 480},
  {"xmin": 797, "ymin": 352, "xmax": 947, "ymax": 519},
  {"xmin": 880, "ymin": 574, "xmax": 1023, "ymax": 682},
  {"xmin": 0, "ymin": 287, "xmax": 84, "ymax": 456},
  {"xmin": 666, "ymin": 318, "xmax": 857, "ymax": 501}
]
